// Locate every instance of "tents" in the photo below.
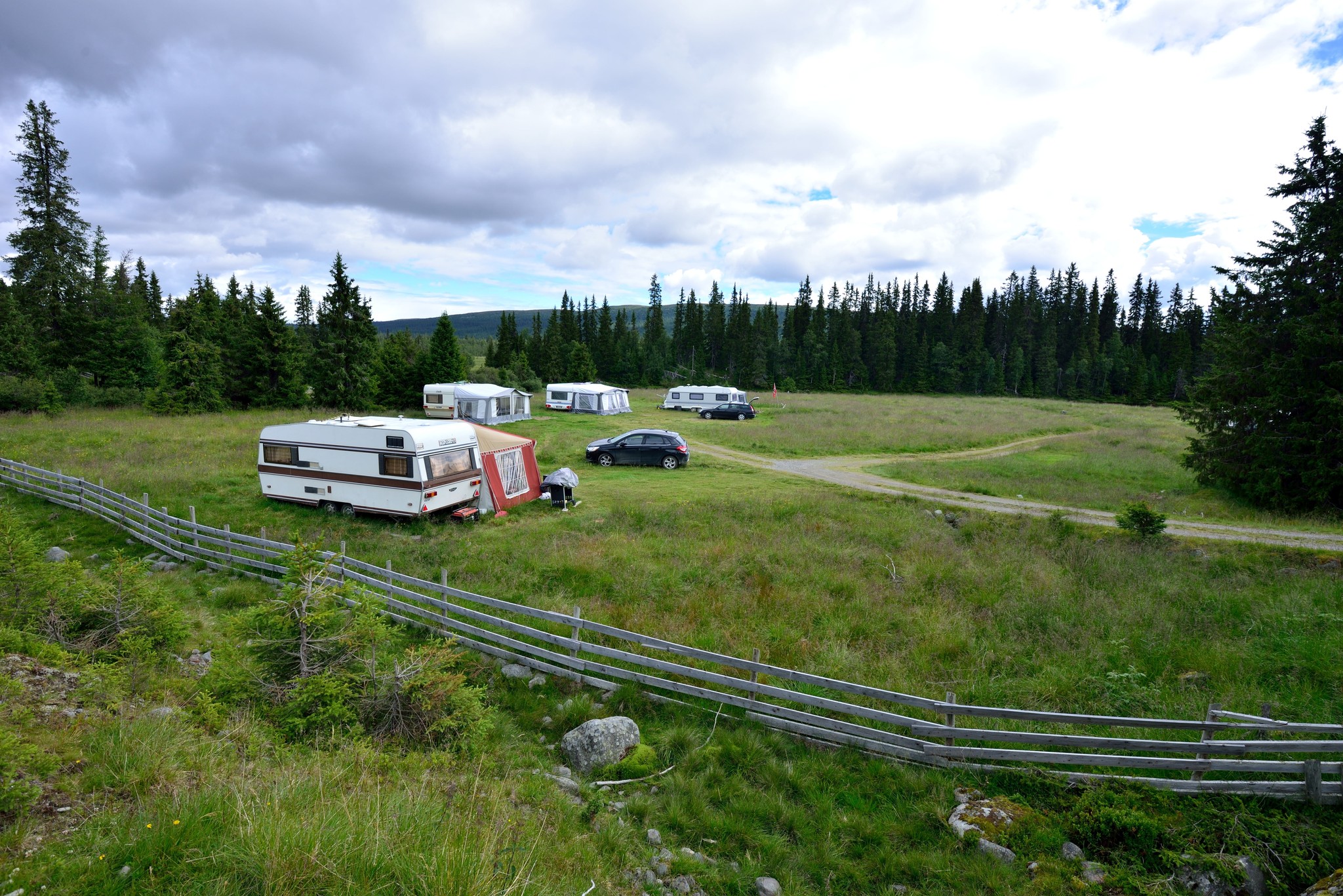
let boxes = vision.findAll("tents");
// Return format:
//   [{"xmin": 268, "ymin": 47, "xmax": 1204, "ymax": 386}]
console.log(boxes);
[
  {"xmin": 711, "ymin": 385, "xmax": 748, "ymax": 403},
  {"xmin": 443, "ymin": 419, "xmax": 543, "ymax": 515},
  {"xmin": 453, "ymin": 386, "xmax": 533, "ymax": 425},
  {"xmin": 570, "ymin": 384, "xmax": 633, "ymax": 416}
]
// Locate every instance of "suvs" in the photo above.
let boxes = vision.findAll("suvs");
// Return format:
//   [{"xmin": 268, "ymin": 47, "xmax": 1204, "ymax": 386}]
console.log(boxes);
[
  {"xmin": 698, "ymin": 397, "xmax": 759, "ymax": 420},
  {"xmin": 585, "ymin": 428, "xmax": 690, "ymax": 470}
]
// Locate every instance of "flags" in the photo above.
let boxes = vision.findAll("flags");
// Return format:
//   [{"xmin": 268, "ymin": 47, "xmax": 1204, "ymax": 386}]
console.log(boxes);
[{"xmin": 773, "ymin": 385, "xmax": 776, "ymax": 398}]
